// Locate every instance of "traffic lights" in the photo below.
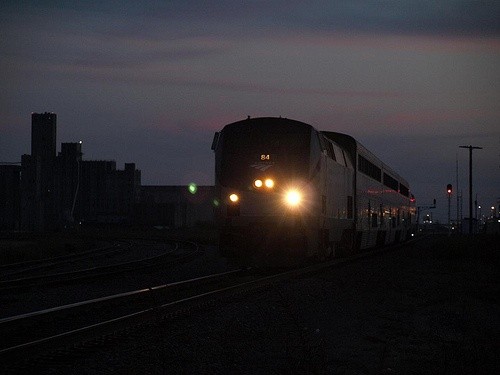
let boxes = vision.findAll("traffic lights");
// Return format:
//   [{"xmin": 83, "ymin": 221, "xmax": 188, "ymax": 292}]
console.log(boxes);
[{"xmin": 446, "ymin": 183, "xmax": 454, "ymax": 194}]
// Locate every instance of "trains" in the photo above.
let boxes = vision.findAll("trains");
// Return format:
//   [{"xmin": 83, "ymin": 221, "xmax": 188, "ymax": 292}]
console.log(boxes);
[{"xmin": 210, "ymin": 114, "xmax": 417, "ymax": 257}]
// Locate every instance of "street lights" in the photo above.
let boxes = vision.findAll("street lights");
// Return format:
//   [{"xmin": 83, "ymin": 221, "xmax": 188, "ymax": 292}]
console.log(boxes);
[
  {"xmin": 478, "ymin": 205, "xmax": 482, "ymax": 217},
  {"xmin": 490, "ymin": 205, "xmax": 495, "ymax": 218}
]
